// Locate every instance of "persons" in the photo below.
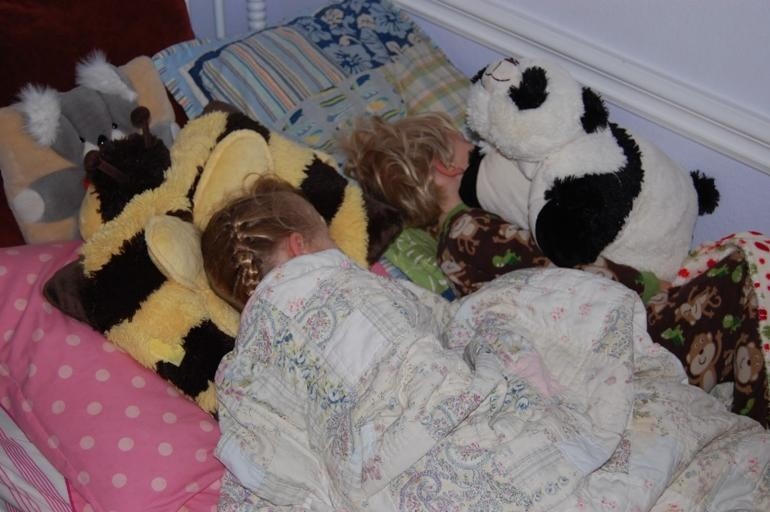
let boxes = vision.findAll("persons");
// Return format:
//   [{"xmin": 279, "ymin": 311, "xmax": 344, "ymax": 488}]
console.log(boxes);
[
  {"xmin": 200, "ymin": 169, "xmax": 345, "ymax": 314},
  {"xmin": 351, "ymin": 111, "xmax": 770, "ymax": 431}
]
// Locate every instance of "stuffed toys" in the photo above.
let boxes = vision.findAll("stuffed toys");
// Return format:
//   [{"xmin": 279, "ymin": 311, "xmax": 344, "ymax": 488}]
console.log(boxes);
[
  {"xmin": 40, "ymin": 97, "xmax": 403, "ymax": 425},
  {"xmin": 456, "ymin": 56, "xmax": 720, "ymax": 284},
  {"xmin": 6, "ymin": 49, "xmax": 181, "ymax": 225}
]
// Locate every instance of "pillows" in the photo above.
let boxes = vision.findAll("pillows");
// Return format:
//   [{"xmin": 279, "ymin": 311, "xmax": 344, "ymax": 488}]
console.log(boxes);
[
  {"xmin": 0, "ymin": 237, "xmax": 223, "ymax": 510},
  {"xmin": 151, "ymin": 1, "xmax": 471, "ymax": 163},
  {"xmin": 1, "ymin": 55, "xmax": 176, "ymax": 244}
]
